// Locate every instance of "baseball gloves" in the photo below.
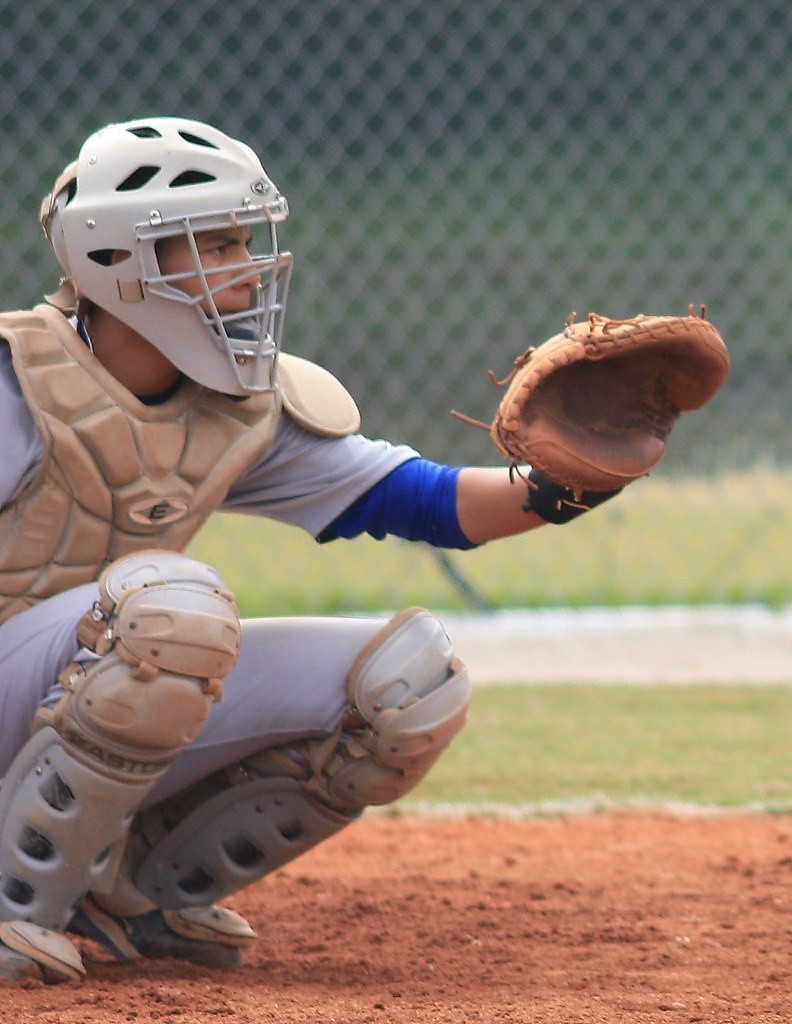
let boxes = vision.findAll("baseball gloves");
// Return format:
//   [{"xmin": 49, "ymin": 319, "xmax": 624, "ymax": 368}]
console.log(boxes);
[{"xmin": 490, "ymin": 314, "xmax": 731, "ymax": 498}]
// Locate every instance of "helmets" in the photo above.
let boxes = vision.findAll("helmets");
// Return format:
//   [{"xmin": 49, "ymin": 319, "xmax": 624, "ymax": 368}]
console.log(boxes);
[{"xmin": 41, "ymin": 118, "xmax": 290, "ymax": 397}]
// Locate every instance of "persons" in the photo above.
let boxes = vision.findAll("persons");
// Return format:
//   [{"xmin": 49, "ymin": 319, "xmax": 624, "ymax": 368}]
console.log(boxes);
[{"xmin": 0, "ymin": 117, "xmax": 729, "ymax": 986}]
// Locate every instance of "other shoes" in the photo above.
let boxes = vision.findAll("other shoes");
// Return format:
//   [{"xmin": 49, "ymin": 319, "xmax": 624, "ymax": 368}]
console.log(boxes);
[
  {"xmin": 0, "ymin": 919, "xmax": 87, "ymax": 985},
  {"xmin": 67, "ymin": 896, "xmax": 257, "ymax": 970}
]
